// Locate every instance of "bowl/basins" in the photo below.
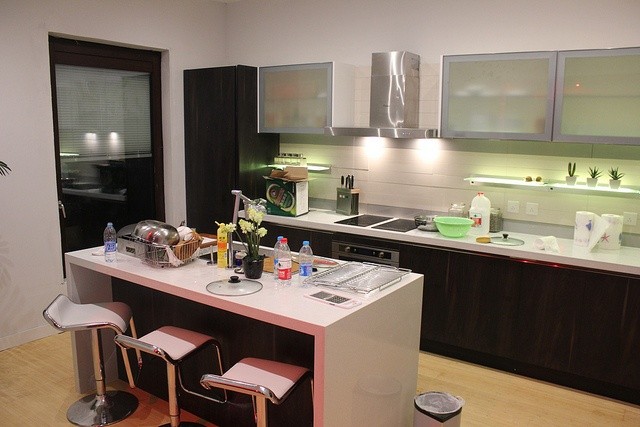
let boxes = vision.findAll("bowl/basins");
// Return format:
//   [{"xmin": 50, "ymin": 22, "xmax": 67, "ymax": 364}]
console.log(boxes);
[
  {"xmin": 131, "ymin": 218, "xmax": 196, "ymax": 260},
  {"xmin": 434, "ymin": 216, "xmax": 474, "ymax": 238}
]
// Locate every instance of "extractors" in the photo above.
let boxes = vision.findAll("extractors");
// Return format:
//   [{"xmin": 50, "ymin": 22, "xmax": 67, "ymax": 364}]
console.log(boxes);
[{"xmin": 334, "ymin": 50, "xmax": 439, "ymax": 141}]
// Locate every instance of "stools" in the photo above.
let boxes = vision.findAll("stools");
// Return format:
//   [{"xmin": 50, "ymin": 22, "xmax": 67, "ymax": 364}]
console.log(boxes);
[
  {"xmin": 42, "ymin": 293, "xmax": 141, "ymax": 426},
  {"xmin": 113, "ymin": 325, "xmax": 228, "ymax": 427},
  {"xmin": 201, "ymin": 356, "xmax": 313, "ymax": 426}
]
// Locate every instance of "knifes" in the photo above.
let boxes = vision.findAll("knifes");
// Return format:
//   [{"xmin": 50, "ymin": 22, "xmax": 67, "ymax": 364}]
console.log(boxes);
[
  {"xmin": 345, "ymin": 174, "xmax": 354, "ymax": 189},
  {"xmin": 340, "ymin": 175, "xmax": 345, "ymax": 188}
]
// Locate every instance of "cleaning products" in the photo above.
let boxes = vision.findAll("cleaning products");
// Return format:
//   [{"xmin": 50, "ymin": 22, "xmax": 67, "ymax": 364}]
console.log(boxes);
[{"xmin": 216, "ymin": 222, "xmax": 226, "ymax": 267}]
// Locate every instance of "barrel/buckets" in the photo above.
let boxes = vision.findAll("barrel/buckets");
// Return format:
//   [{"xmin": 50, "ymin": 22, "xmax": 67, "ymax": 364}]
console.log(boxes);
[{"xmin": 469, "ymin": 193, "xmax": 491, "ymax": 235}]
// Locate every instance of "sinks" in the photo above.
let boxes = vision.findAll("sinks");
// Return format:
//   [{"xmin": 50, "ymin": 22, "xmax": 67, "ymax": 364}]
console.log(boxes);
[{"xmin": 197, "ymin": 244, "xmax": 291, "ymax": 268}]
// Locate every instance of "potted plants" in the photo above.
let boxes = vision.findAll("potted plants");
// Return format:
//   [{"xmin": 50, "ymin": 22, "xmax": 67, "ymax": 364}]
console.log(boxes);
[
  {"xmin": 586, "ymin": 166, "xmax": 604, "ymax": 187},
  {"xmin": 565, "ymin": 162, "xmax": 578, "ymax": 186},
  {"xmin": 214, "ymin": 208, "xmax": 268, "ymax": 279},
  {"xmin": 608, "ymin": 167, "xmax": 625, "ymax": 189}
]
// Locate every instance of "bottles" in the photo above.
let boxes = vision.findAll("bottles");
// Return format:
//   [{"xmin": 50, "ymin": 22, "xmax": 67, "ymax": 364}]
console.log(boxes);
[
  {"xmin": 103, "ymin": 222, "xmax": 117, "ymax": 264},
  {"xmin": 277, "ymin": 238, "xmax": 292, "ymax": 288},
  {"xmin": 273, "ymin": 236, "xmax": 285, "ymax": 283},
  {"xmin": 489, "ymin": 207, "xmax": 504, "ymax": 233},
  {"xmin": 447, "ymin": 201, "xmax": 469, "ymax": 219},
  {"xmin": 298, "ymin": 240, "xmax": 313, "ymax": 289}
]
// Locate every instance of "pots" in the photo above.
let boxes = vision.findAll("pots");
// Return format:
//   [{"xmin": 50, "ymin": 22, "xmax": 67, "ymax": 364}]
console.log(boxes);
[{"xmin": 414, "ymin": 215, "xmax": 441, "ymax": 232}]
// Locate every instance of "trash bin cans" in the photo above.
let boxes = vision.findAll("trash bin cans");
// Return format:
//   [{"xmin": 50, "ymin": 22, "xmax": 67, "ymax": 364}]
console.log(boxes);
[{"xmin": 413, "ymin": 391, "xmax": 463, "ymax": 426}]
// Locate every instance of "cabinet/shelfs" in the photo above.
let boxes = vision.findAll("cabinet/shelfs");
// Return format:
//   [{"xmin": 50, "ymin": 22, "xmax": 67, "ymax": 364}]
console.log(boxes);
[
  {"xmin": 514, "ymin": 261, "xmax": 640, "ymax": 391},
  {"xmin": 439, "ymin": 51, "xmax": 557, "ymax": 143},
  {"xmin": 256, "ymin": 61, "xmax": 355, "ymax": 137},
  {"xmin": 402, "ymin": 245, "xmax": 522, "ymax": 360},
  {"xmin": 183, "ymin": 64, "xmax": 279, "ymax": 234},
  {"xmin": 552, "ymin": 47, "xmax": 640, "ymax": 146}
]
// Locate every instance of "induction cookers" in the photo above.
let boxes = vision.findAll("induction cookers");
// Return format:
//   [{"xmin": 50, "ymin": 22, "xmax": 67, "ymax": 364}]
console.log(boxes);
[
  {"xmin": 333, "ymin": 214, "xmax": 394, "ymax": 228},
  {"xmin": 371, "ymin": 217, "xmax": 420, "ymax": 234}
]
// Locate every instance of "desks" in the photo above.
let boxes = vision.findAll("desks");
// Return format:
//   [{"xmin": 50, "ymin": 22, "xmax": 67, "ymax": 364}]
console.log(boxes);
[{"xmin": 64, "ymin": 235, "xmax": 425, "ymax": 427}]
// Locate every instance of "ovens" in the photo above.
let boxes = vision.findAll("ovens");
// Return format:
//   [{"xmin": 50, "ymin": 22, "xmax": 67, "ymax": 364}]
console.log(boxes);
[{"xmin": 329, "ymin": 237, "xmax": 400, "ymax": 269}]
